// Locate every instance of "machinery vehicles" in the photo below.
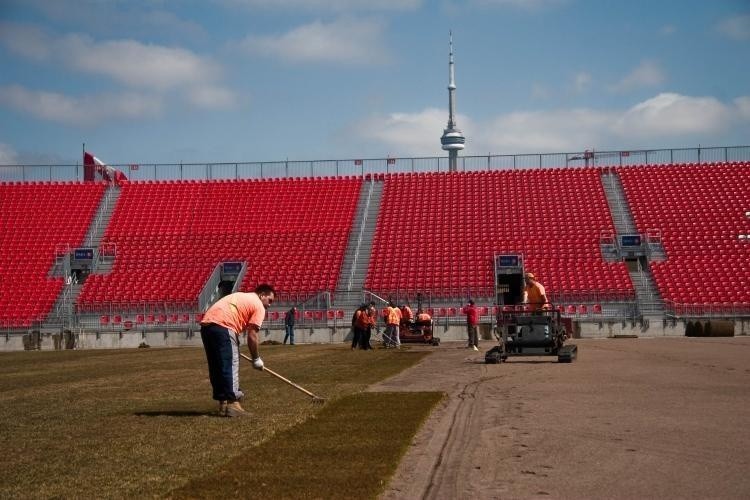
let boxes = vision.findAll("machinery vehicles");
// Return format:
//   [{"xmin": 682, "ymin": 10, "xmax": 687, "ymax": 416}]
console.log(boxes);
[{"xmin": 487, "ymin": 303, "xmax": 579, "ymax": 365}]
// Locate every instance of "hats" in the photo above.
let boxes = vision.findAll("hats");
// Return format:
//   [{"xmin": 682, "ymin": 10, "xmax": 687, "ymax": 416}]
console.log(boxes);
[{"xmin": 523, "ymin": 273, "xmax": 534, "ymax": 278}]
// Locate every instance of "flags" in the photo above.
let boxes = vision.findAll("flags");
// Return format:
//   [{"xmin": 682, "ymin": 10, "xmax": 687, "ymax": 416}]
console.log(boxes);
[{"xmin": 84, "ymin": 153, "xmax": 127, "ymax": 184}]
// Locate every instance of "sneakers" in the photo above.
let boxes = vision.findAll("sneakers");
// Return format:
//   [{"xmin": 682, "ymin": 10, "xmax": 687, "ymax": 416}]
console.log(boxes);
[{"xmin": 218, "ymin": 401, "xmax": 246, "ymax": 417}]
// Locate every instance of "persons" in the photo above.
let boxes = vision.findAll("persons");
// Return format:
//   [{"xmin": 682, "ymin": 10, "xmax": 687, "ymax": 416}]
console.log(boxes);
[
  {"xmin": 284, "ymin": 306, "xmax": 296, "ymax": 344},
  {"xmin": 351, "ymin": 301, "xmax": 431, "ymax": 350},
  {"xmin": 463, "ymin": 299, "xmax": 478, "ymax": 348},
  {"xmin": 522, "ymin": 273, "xmax": 548, "ymax": 316},
  {"xmin": 200, "ymin": 284, "xmax": 276, "ymax": 417}
]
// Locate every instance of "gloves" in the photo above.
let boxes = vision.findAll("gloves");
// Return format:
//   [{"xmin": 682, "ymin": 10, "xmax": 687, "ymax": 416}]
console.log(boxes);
[{"xmin": 251, "ymin": 356, "xmax": 266, "ymax": 371}]
